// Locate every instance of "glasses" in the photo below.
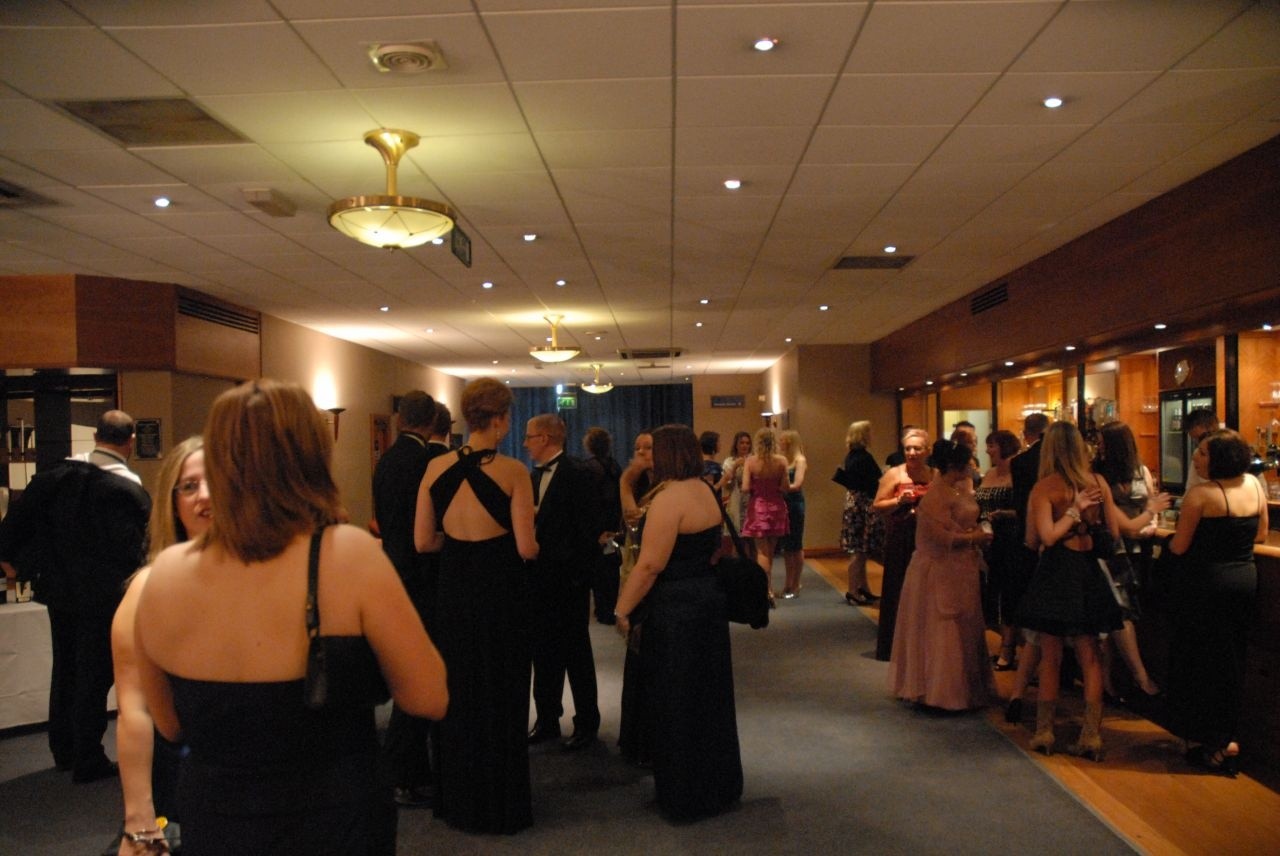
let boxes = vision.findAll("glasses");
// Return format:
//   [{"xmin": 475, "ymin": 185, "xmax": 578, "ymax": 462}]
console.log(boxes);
[
  {"xmin": 173, "ymin": 479, "xmax": 208, "ymax": 496},
  {"xmin": 524, "ymin": 432, "xmax": 553, "ymax": 440}
]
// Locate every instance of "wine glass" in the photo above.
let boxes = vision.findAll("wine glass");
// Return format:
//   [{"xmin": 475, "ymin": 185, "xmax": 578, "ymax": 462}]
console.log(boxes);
[{"xmin": 624, "ymin": 508, "xmax": 640, "ymax": 550}]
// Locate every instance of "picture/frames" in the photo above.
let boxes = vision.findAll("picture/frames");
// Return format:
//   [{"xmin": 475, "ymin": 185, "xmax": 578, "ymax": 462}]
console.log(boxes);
[{"xmin": 371, "ymin": 414, "xmax": 392, "ymax": 471}]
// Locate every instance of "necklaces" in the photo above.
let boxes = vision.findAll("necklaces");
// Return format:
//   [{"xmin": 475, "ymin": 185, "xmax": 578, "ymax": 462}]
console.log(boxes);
[{"xmin": 946, "ymin": 483, "xmax": 969, "ymax": 500}]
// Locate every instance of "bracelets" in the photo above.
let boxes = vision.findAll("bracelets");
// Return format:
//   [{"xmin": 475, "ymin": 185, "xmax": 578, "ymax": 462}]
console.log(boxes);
[
  {"xmin": 1066, "ymin": 508, "xmax": 1080, "ymax": 521},
  {"xmin": 120, "ymin": 816, "xmax": 179, "ymax": 846},
  {"xmin": 615, "ymin": 609, "xmax": 624, "ymax": 618}
]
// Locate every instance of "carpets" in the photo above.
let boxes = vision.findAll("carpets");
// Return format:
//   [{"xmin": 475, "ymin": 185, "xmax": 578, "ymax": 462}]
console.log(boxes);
[{"xmin": 0, "ymin": 553, "xmax": 1136, "ymax": 856}]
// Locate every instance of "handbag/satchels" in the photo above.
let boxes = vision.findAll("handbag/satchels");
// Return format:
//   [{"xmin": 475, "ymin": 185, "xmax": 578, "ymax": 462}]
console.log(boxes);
[
  {"xmin": 699, "ymin": 476, "xmax": 769, "ymax": 630},
  {"xmin": 304, "ymin": 525, "xmax": 393, "ymax": 708},
  {"xmin": 831, "ymin": 448, "xmax": 864, "ymax": 493}
]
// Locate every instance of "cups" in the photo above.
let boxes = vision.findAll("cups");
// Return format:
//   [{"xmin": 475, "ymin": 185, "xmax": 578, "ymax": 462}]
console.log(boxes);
[
  {"xmin": 977, "ymin": 521, "xmax": 992, "ymax": 533},
  {"xmin": 16, "ymin": 581, "xmax": 31, "ymax": 602},
  {"xmin": 1152, "ymin": 512, "xmax": 1167, "ymax": 529},
  {"xmin": 1267, "ymin": 382, "xmax": 1280, "ymax": 402}
]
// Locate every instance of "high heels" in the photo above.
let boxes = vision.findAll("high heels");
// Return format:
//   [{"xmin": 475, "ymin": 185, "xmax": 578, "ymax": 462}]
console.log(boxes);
[
  {"xmin": 1029, "ymin": 731, "xmax": 1055, "ymax": 756},
  {"xmin": 994, "ymin": 641, "xmax": 1017, "ymax": 671},
  {"xmin": 1103, "ymin": 687, "xmax": 1129, "ymax": 705},
  {"xmin": 1130, "ymin": 676, "xmax": 1165, "ymax": 703},
  {"xmin": 1201, "ymin": 742, "xmax": 1241, "ymax": 776},
  {"xmin": 1177, "ymin": 740, "xmax": 1211, "ymax": 770},
  {"xmin": 1064, "ymin": 734, "xmax": 1102, "ymax": 762},
  {"xmin": 845, "ymin": 587, "xmax": 880, "ymax": 606},
  {"xmin": 1003, "ymin": 697, "xmax": 1024, "ymax": 725}
]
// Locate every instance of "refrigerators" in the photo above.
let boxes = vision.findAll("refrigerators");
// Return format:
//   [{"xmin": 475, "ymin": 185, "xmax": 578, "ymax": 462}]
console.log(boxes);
[{"xmin": 1157, "ymin": 384, "xmax": 1217, "ymax": 495}]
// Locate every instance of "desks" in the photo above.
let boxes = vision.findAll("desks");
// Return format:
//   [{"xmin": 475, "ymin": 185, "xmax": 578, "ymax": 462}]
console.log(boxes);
[{"xmin": 0, "ymin": 587, "xmax": 119, "ymax": 728}]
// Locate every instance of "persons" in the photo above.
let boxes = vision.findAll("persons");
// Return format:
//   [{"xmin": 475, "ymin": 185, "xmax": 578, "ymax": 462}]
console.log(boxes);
[
  {"xmin": 840, "ymin": 421, "xmax": 881, "ymax": 605},
  {"xmin": 875, "ymin": 406, "xmax": 1268, "ymax": 768},
  {"xmin": 427, "ymin": 404, "xmax": 807, "ymax": 626},
  {"xmin": 377, "ymin": 391, "xmax": 435, "ymax": 806},
  {"xmin": 102, "ymin": 435, "xmax": 212, "ymax": 856},
  {"xmin": 526, "ymin": 415, "xmax": 602, "ymax": 750},
  {"xmin": 614, "ymin": 426, "xmax": 742, "ymax": 825},
  {"xmin": 0, "ymin": 410, "xmax": 154, "ymax": 783},
  {"xmin": 414, "ymin": 377, "xmax": 540, "ymax": 835},
  {"xmin": 133, "ymin": 378, "xmax": 447, "ymax": 856}
]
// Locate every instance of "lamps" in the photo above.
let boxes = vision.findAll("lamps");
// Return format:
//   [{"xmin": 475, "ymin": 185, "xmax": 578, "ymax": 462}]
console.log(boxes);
[
  {"xmin": 581, "ymin": 364, "xmax": 613, "ymax": 395},
  {"xmin": 527, "ymin": 314, "xmax": 580, "ymax": 364},
  {"xmin": 760, "ymin": 412, "xmax": 777, "ymax": 429},
  {"xmin": 328, "ymin": 405, "xmax": 346, "ymax": 441},
  {"xmin": 326, "ymin": 124, "xmax": 460, "ymax": 255}
]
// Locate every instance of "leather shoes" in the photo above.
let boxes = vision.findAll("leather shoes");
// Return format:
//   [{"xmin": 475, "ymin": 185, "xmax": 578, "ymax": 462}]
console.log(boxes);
[
  {"xmin": 560, "ymin": 726, "xmax": 599, "ymax": 753},
  {"xmin": 393, "ymin": 780, "xmax": 431, "ymax": 807},
  {"xmin": 53, "ymin": 748, "xmax": 120, "ymax": 784},
  {"xmin": 528, "ymin": 720, "xmax": 561, "ymax": 744}
]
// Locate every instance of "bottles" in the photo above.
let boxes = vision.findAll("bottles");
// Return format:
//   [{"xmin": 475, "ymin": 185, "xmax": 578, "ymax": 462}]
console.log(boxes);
[
  {"xmin": 1255, "ymin": 418, "xmax": 1280, "ymax": 502},
  {"xmin": 1172, "ymin": 407, "xmax": 1182, "ymax": 430}
]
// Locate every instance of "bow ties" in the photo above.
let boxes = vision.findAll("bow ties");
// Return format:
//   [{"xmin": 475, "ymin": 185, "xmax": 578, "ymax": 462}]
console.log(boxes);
[{"xmin": 533, "ymin": 452, "xmax": 567, "ymax": 471}]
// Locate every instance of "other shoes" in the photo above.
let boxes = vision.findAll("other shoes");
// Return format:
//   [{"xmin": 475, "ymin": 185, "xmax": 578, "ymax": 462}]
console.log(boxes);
[{"xmin": 780, "ymin": 584, "xmax": 803, "ymax": 599}]
